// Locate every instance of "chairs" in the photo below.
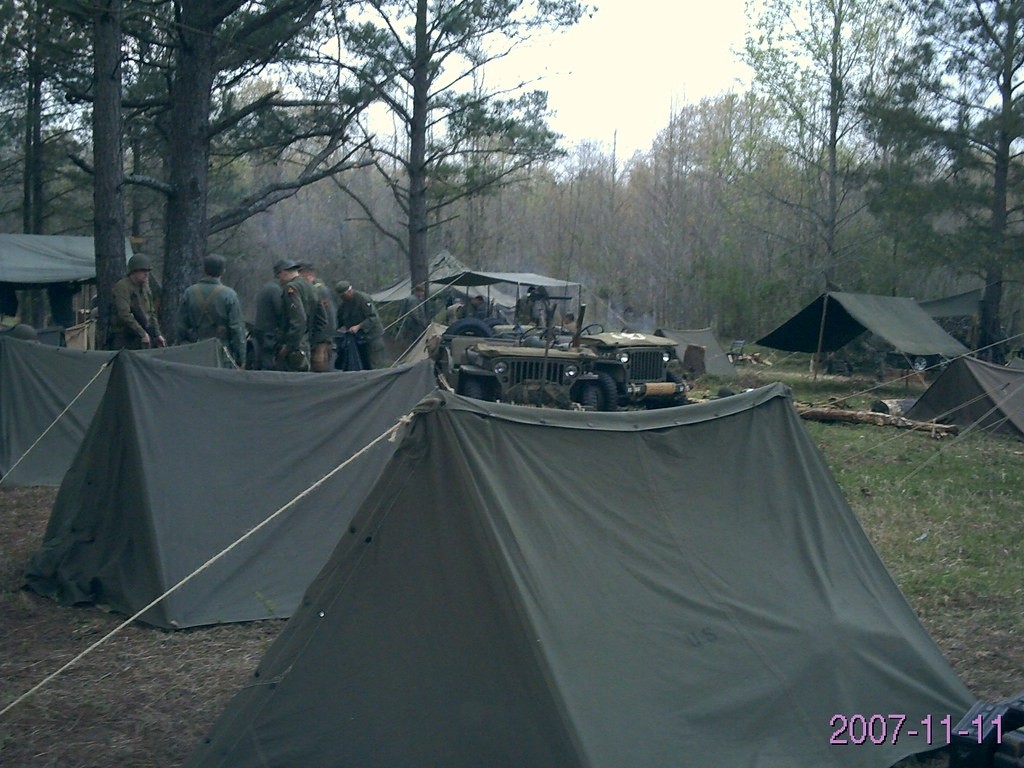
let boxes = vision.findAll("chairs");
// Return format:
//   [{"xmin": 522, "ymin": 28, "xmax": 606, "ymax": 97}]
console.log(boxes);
[{"xmin": 726, "ymin": 339, "xmax": 746, "ymax": 367}]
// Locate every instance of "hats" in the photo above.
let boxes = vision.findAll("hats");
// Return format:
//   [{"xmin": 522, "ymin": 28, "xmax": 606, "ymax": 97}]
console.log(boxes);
[
  {"xmin": 296, "ymin": 259, "xmax": 314, "ymax": 270},
  {"xmin": 272, "ymin": 258, "xmax": 301, "ymax": 276}
]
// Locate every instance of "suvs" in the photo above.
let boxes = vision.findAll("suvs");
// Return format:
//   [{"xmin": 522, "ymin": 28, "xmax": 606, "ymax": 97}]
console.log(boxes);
[{"xmin": 420, "ymin": 317, "xmax": 688, "ymax": 414}]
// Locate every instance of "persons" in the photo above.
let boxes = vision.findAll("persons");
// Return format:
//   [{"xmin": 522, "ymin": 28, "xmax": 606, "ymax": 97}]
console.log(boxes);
[{"xmin": 0, "ymin": 252, "xmax": 578, "ymax": 373}]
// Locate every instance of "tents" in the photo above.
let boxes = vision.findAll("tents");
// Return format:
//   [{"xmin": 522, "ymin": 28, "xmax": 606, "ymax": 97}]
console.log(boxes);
[
  {"xmin": 188, "ymin": 393, "xmax": 979, "ymax": 768},
  {"xmin": 753, "ymin": 282, "xmax": 1024, "ymax": 441},
  {"xmin": 370, "ymin": 251, "xmax": 740, "ymax": 384},
  {"xmin": 0, "ymin": 335, "xmax": 439, "ymax": 630},
  {"xmin": 0, "ymin": 231, "xmax": 134, "ymax": 283}
]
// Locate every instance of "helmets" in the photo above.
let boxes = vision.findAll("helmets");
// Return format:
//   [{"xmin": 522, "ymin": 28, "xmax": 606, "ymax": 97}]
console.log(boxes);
[
  {"xmin": 338, "ymin": 278, "xmax": 352, "ymax": 294},
  {"xmin": 125, "ymin": 252, "xmax": 153, "ymax": 275}
]
